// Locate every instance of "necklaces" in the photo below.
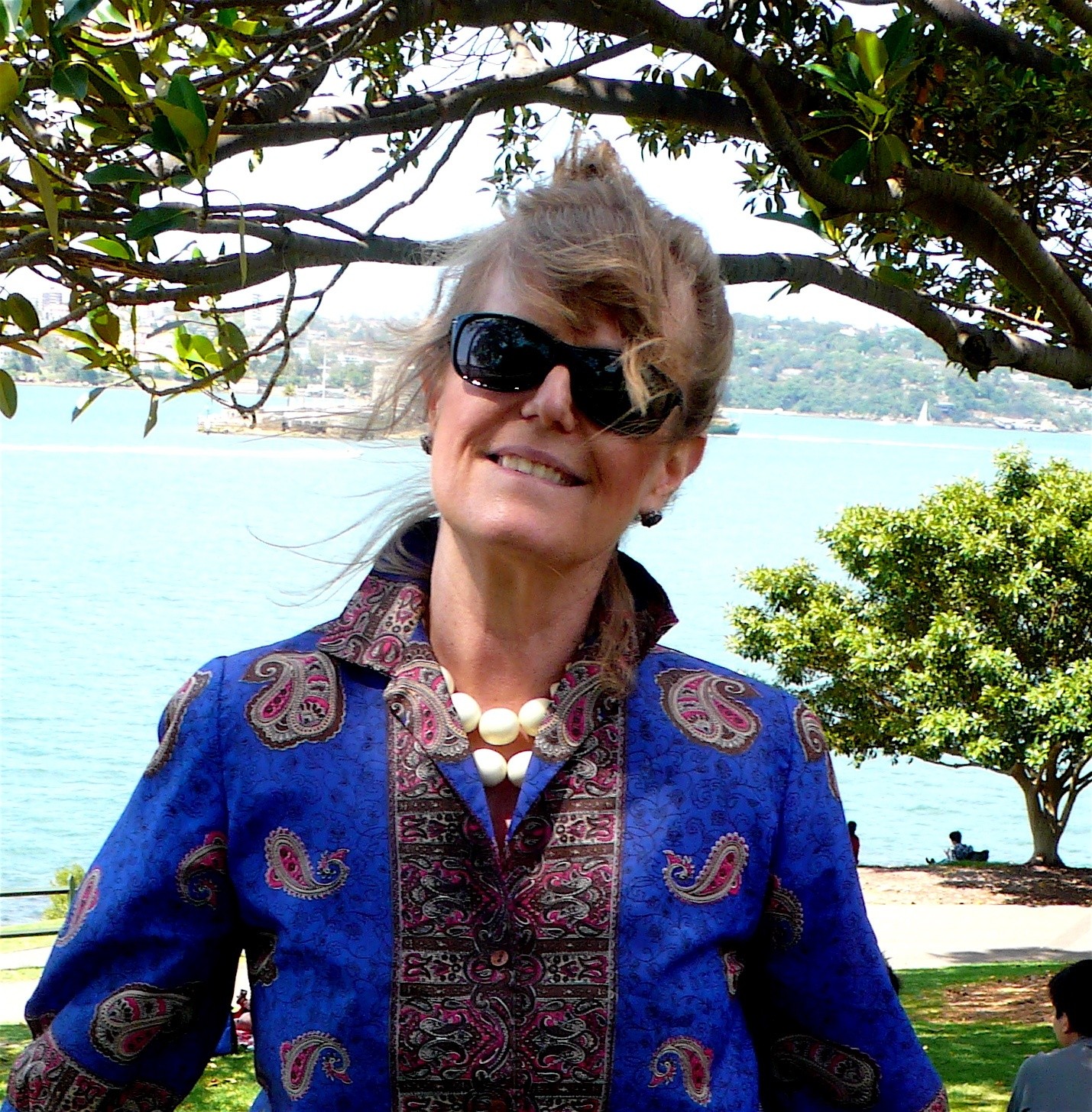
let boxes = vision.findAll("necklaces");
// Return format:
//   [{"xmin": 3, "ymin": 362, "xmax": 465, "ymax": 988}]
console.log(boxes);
[{"xmin": 439, "ymin": 663, "xmax": 571, "ymax": 786}]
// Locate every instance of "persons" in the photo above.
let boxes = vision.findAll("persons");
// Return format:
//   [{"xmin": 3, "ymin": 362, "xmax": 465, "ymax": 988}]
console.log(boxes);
[
  {"xmin": 1006, "ymin": 960, "xmax": 1092, "ymax": 1112},
  {"xmin": 926, "ymin": 831, "xmax": 973, "ymax": 864},
  {"xmin": 848, "ymin": 821, "xmax": 859, "ymax": 861},
  {"xmin": 0, "ymin": 142, "xmax": 950, "ymax": 1111}
]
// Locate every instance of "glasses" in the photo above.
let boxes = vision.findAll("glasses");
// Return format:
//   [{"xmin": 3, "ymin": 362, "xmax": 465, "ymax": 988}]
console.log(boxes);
[{"xmin": 446, "ymin": 312, "xmax": 691, "ymax": 437}]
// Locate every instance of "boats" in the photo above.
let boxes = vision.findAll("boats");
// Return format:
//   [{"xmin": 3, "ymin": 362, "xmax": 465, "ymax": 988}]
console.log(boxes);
[{"xmin": 705, "ymin": 410, "xmax": 740, "ymax": 435}]
